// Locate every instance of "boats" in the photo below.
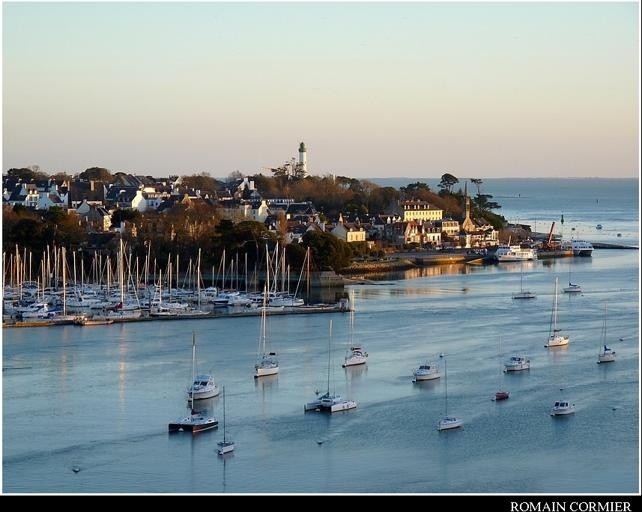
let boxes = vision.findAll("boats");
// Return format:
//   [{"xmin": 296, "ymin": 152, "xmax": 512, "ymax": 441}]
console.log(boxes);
[
  {"xmin": 69, "ymin": 465, "xmax": 83, "ymax": 472},
  {"xmin": 316, "ymin": 439, "xmax": 325, "ymax": 447},
  {"xmin": 72, "ymin": 317, "xmax": 118, "ymax": 327},
  {"xmin": 469, "ymin": 222, "xmax": 594, "ymax": 260},
  {"xmin": 595, "ymin": 223, "xmax": 602, "ymax": 229},
  {"xmin": 403, "ymin": 276, "xmax": 614, "ymax": 431}
]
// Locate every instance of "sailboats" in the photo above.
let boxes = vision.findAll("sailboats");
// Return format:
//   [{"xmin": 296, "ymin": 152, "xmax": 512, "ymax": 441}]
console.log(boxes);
[
  {"xmin": 168, "ymin": 331, "xmax": 219, "ymax": 435},
  {"xmin": 344, "ymin": 289, "xmax": 369, "ymax": 368},
  {"xmin": 303, "ymin": 319, "xmax": 356, "ymax": 416},
  {"xmin": 252, "ymin": 285, "xmax": 279, "ymax": 379},
  {"xmin": 2, "ymin": 238, "xmax": 335, "ymax": 320},
  {"xmin": 216, "ymin": 385, "xmax": 234, "ymax": 455}
]
